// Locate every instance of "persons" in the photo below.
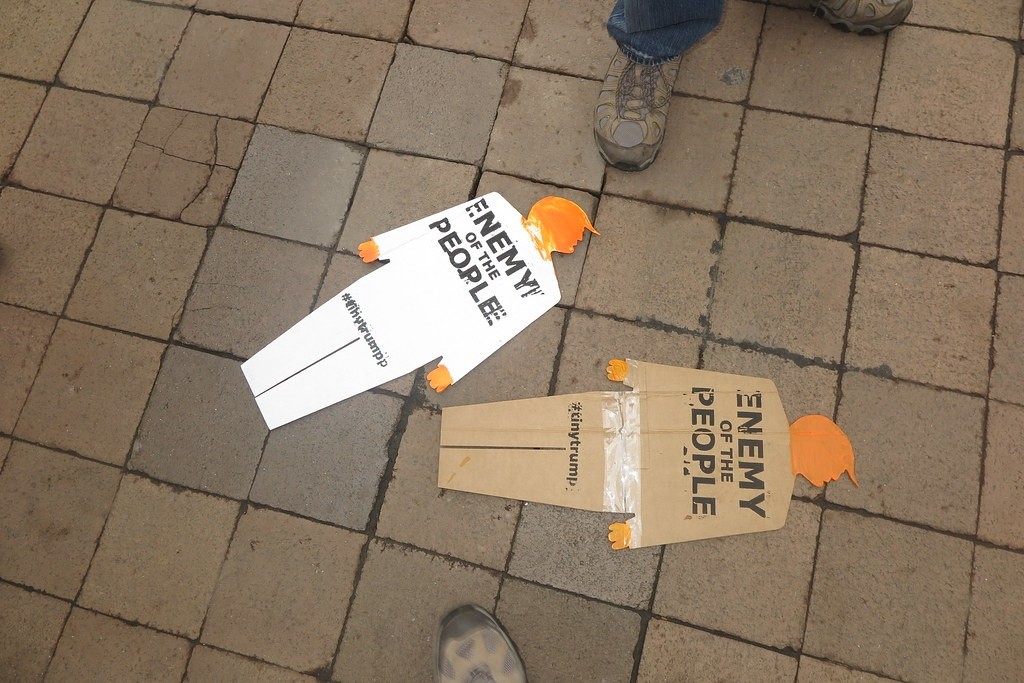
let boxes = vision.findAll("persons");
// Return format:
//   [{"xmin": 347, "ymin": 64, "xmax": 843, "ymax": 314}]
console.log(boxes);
[{"xmin": 591, "ymin": 1, "xmax": 912, "ymax": 172}]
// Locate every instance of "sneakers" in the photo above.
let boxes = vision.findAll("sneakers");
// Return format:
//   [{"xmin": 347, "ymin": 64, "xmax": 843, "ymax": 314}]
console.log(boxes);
[
  {"xmin": 592, "ymin": 48, "xmax": 685, "ymax": 172},
  {"xmin": 748, "ymin": 0, "xmax": 914, "ymax": 37}
]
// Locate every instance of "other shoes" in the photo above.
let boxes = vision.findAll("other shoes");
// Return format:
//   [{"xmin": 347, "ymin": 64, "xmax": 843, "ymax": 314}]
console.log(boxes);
[{"xmin": 434, "ymin": 604, "xmax": 529, "ymax": 682}]
